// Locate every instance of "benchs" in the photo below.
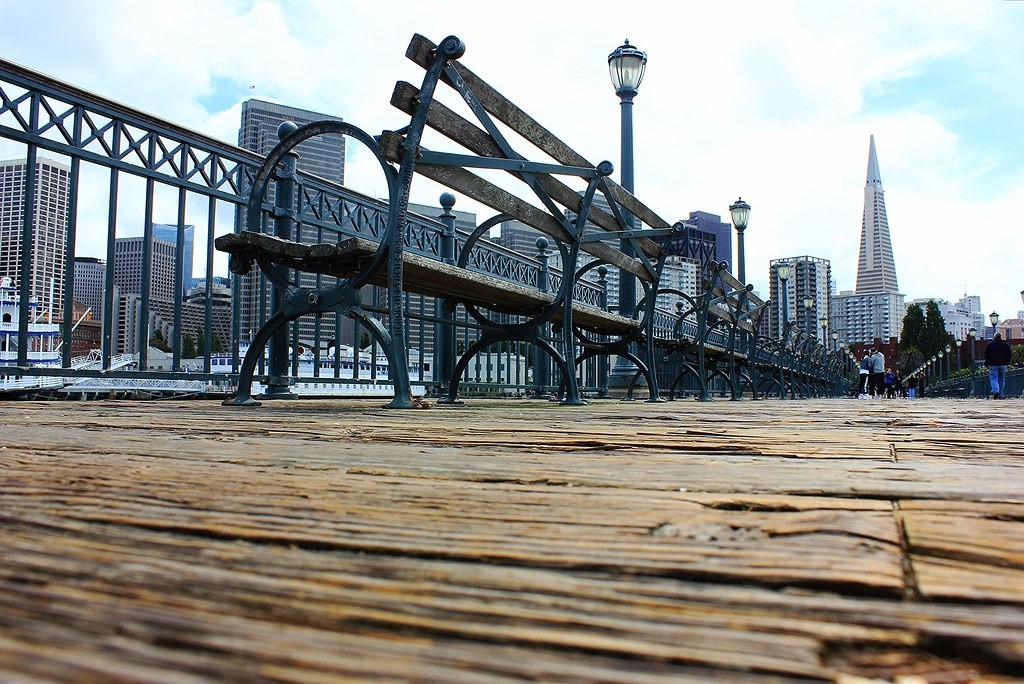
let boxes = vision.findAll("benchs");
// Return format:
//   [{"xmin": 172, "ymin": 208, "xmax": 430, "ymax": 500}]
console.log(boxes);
[
  {"xmin": 214, "ymin": 33, "xmax": 691, "ymax": 409},
  {"xmin": 734, "ymin": 321, "xmax": 815, "ymax": 401},
  {"xmin": 785, "ymin": 343, "xmax": 847, "ymax": 401},
  {"xmin": 618, "ymin": 259, "xmax": 774, "ymax": 403}
]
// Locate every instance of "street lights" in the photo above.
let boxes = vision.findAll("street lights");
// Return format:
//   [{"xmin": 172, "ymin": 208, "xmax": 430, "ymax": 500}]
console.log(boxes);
[
  {"xmin": 989, "ymin": 311, "xmax": 1000, "ymax": 336},
  {"xmin": 969, "ymin": 327, "xmax": 979, "ymax": 398},
  {"xmin": 839, "ymin": 338, "xmax": 845, "ymax": 363},
  {"xmin": 819, "ymin": 315, "xmax": 830, "ymax": 398},
  {"xmin": 931, "ymin": 355, "xmax": 937, "ymax": 382},
  {"xmin": 830, "ymin": 329, "xmax": 838, "ymax": 353},
  {"xmin": 927, "ymin": 360, "xmax": 932, "ymax": 385},
  {"xmin": 848, "ymin": 351, "xmax": 854, "ymax": 375},
  {"xmin": 919, "ymin": 365, "xmax": 923, "ymax": 387},
  {"xmin": 955, "ymin": 338, "xmax": 963, "ymax": 377},
  {"xmin": 776, "ymin": 258, "xmax": 799, "ymax": 400},
  {"xmin": 923, "ymin": 362, "xmax": 927, "ymax": 388},
  {"xmin": 802, "ymin": 293, "xmax": 813, "ymax": 399},
  {"xmin": 916, "ymin": 367, "xmax": 920, "ymax": 388},
  {"xmin": 938, "ymin": 351, "xmax": 944, "ymax": 382},
  {"xmin": 945, "ymin": 343, "xmax": 951, "ymax": 380},
  {"xmin": 843, "ymin": 346, "xmax": 850, "ymax": 379},
  {"xmin": 607, "ymin": 37, "xmax": 652, "ymax": 398},
  {"xmin": 728, "ymin": 197, "xmax": 755, "ymax": 391}
]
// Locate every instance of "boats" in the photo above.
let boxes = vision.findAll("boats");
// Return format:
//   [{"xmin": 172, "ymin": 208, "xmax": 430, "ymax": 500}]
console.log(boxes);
[{"xmin": 0, "ymin": 271, "xmax": 550, "ymax": 397}]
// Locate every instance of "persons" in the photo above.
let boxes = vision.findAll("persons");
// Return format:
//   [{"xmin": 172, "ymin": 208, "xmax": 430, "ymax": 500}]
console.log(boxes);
[
  {"xmin": 985, "ymin": 331, "xmax": 1012, "ymax": 399},
  {"xmin": 857, "ymin": 348, "xmax": 925, "ymax": 400}
]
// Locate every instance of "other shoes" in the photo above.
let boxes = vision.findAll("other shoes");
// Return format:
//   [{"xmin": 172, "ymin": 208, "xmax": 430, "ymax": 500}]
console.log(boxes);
[
  {"xmin": 1000, "ymin": 396, "xmax": 1006, "ymax": 399},
  {"xmin": 993, "ymin": 392, "xmax": 999, "ymax": 399},
  {"xmin": 859, "ymin": 394, "xmax": 882, "ymax": 400}
]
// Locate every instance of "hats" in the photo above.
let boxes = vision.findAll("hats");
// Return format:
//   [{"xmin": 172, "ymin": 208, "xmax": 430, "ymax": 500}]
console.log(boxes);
[{"xmin": 871, "ymin": 348, "xmax": 877, "ymax": 354}]
